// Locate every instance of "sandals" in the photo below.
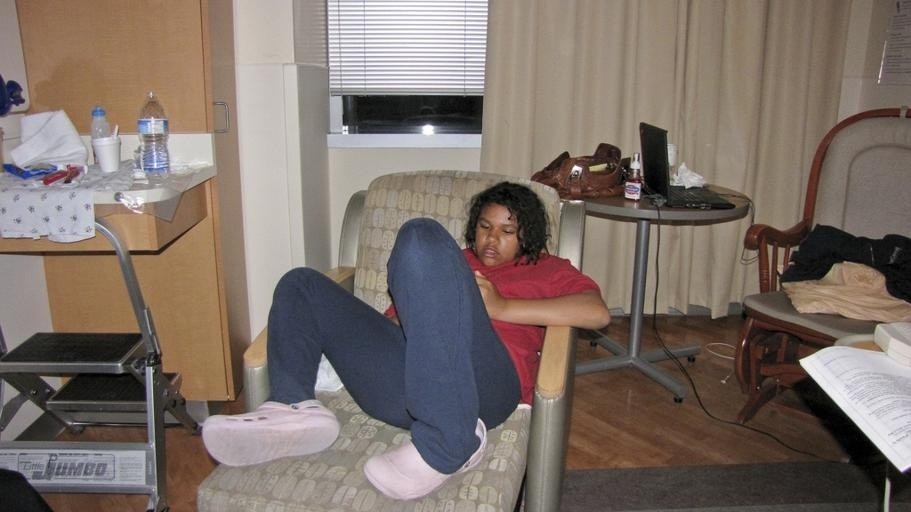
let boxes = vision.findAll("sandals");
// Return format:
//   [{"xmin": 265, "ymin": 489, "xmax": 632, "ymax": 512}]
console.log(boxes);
[
  {"xmin": 364, "ymin": 417, "xmax": 488, "ymax": 500},
  {"xmin": 202, "ymin": 399, "xmax": 340, "ymax": 467}
]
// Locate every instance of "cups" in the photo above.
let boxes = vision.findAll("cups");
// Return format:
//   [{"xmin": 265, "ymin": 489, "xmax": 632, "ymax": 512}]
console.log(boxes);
[{"xmin": 92, "ymin": 136, "xmax": 122, "ymax": 173}]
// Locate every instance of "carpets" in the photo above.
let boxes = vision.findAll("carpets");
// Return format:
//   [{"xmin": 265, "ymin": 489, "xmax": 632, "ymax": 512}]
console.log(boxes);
[{"xmin": 557, "ymin": 461, "xmax": 911, "ymax": 512}]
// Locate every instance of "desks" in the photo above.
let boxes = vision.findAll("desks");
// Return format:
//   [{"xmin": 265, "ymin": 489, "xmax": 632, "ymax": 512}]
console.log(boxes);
[
  {"xmin": 576, "ymin": 181, "xmax": 752, "ymax": 404},
  {"xmin": 834, "ymin": 331, "xmax": 911, "ymax": 512},
  {"xmin": 0, "ymin": 128, "xmax": 217, "ymax": 254}
]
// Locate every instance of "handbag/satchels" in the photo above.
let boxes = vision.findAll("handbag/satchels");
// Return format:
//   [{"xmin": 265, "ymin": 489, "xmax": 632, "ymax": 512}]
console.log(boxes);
[
  {"xmin": 531, "ymin": 143, "xmax": 628, "ymax": 201},
  {"xmin": 781, "ymin": 224, "xmax": 911, "ymax": 306}
]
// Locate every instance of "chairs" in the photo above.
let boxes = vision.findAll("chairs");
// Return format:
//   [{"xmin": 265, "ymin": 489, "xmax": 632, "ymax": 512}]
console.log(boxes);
[{"xmin": 733, "ymin": 105, "xmax": 911, "ymax": 424}]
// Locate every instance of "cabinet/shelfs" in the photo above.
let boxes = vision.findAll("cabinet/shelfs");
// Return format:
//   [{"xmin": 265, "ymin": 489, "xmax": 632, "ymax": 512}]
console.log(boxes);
[{"xmin": 12, "ymin": 1, "xmax": 248, "ymax": 403}]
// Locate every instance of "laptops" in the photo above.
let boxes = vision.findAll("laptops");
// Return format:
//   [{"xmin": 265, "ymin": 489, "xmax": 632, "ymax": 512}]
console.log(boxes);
[{"xmin": 639, "ymin": 123, "xmax": 736, "ymax": 210}]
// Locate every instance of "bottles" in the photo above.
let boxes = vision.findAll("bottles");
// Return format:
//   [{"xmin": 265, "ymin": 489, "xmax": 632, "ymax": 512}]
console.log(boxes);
[
  {"xmin": 88, "ymin": 105, "xmax": 111, "ymax": 164},
  {"xmin": 623, "ymin": 152, "xmax": 644, "ymax": 201},
  {"xmin": 136, "ymin": 89, "xmax": 171, "ymax": 175}
]
